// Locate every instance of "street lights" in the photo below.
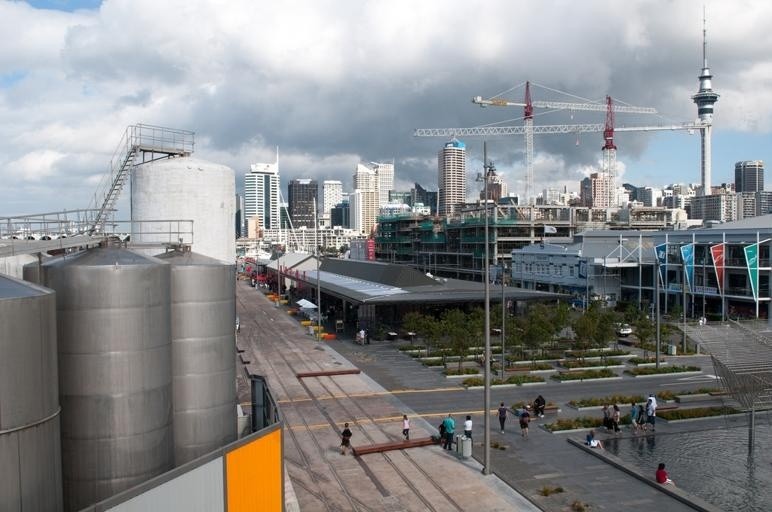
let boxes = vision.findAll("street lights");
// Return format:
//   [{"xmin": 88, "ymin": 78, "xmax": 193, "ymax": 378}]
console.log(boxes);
[{"xmin": 476, "ymin": 140, "xmax": 496, "ymax": 474}]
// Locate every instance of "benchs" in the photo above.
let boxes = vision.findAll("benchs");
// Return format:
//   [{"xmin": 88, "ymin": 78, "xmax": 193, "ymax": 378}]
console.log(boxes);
[
  {"xmin": 645, "ymin": 405, "xmax": 679, "ymax": 411},
  {"xmin": 526, "ymin": 405, "xmax": 559, "ymax": 417},
  {"xmin": 297, "ymin": 369, "xmax": 360, "ymax": 378},
  {"xmin": 352, "ymin": 435, "xmax": 446, "ymax": 457}
]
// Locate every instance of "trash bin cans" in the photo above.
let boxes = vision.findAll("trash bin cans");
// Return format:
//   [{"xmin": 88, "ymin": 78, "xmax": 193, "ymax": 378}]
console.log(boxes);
[
  {"xmin": 455, "ymin": 434, "xmax": 472, "ymax": 457},
  {"xmin": 335, "ymin": 320, "xmax": 345, "ymax": 334},
  {"xmin": 668, "ymin": 345, "xmax": 677, "ymax": 356}
]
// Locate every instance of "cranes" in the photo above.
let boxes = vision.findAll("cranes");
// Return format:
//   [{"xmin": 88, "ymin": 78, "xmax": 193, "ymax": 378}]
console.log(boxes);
[{"xmin": 412, "ymin": 80, "xmax": 712, "ymax": 207}]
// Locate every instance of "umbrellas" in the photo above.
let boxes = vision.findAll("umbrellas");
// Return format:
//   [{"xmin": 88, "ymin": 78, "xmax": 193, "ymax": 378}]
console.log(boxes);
[{"xmin": 296, "ymin": 299, "xmax": 318, "ymax": 308}]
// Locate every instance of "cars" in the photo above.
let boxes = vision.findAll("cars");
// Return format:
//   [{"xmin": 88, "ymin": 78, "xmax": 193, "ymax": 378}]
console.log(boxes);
[{"xmin": 615, "ymin": 323, "xmax": 632, "ymax": 337}]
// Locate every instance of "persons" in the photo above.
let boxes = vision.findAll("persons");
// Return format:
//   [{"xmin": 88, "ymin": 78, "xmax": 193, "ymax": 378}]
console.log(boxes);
[
  {"xmin": 339, "ymin": 423, "xmax": 352, "ymax": 456},
  {"xmin": 519, "ymin": 406, "xmax": 531, "ymax": 440},
  {"xmin": 496, "ymin": 401, "xmax": 508, "ymax": 435},
  {"xmin": 586, "ymin": 430, "xmax": 604, "ymax": 449},
  {"xmin": 601, "ymin": 402, "xmax": 624, "ymax": 436},
  {"xmin": 463, "ymin": 415, "xmax": 473, "ymax": 438},
  {"xmin": 403, "ymin": 414, "xmax": 410, "ymax": 440},
  {"xmin": 439, "ymin": 413, "xmax": 455, "ymax": 450},
  {"xmin": 534, "ymin": 394, "xmax": 545, "ymax": 418},
  {"xmin": 359, "ymin": 327, "xmax": 370, "ymax": 346},
  {"xmin": 630, "ymin": 393, "xmax": 657, "ymax": 435},
  {"xmin": 656, "ymin": 463, "xmax": 676, "ymax": 486}
]
